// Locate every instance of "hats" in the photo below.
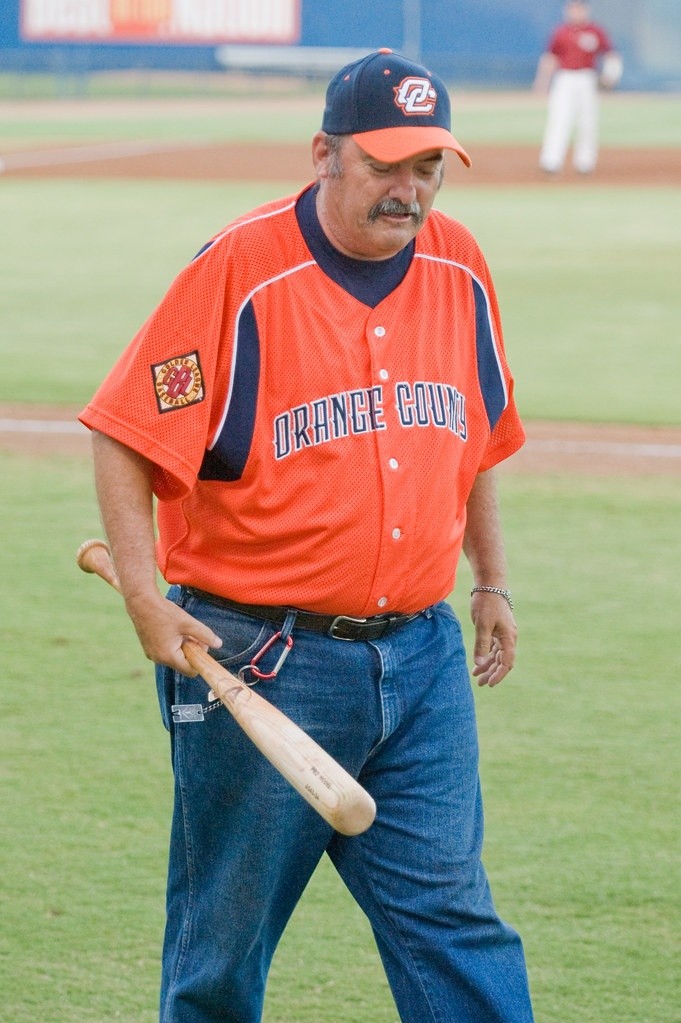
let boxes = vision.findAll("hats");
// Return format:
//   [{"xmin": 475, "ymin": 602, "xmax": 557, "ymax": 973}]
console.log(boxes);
[{"xmin": 322, "ymin": 48, "xmax": 471, "ymax": 169}]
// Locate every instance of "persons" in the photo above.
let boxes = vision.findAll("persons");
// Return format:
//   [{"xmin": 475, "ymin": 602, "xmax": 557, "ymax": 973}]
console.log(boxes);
[
  {"xmin": 531, "ymin": 0, "xmax": 623, "ymax": 176},
  {"xmin": 76, "ymin": 48, "xmax": 536, "ymax": 1023}
]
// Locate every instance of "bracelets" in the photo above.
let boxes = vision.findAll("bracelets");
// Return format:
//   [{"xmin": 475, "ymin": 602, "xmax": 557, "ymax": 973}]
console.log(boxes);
[{"xmin": 471, "ymin": 586, "xmax": 514, "ymax": 612}]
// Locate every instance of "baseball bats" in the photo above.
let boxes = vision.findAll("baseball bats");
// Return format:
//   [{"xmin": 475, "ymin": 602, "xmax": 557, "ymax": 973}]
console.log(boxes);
[{"xmin": 77, "ymin": 539, "xmax": 378, "ymax": 837}]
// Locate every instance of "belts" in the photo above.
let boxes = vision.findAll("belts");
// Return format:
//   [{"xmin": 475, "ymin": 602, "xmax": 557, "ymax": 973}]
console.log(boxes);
[{"xmin": 173, "ymin": 574, "xmax": 431, "ymax": 642}]
